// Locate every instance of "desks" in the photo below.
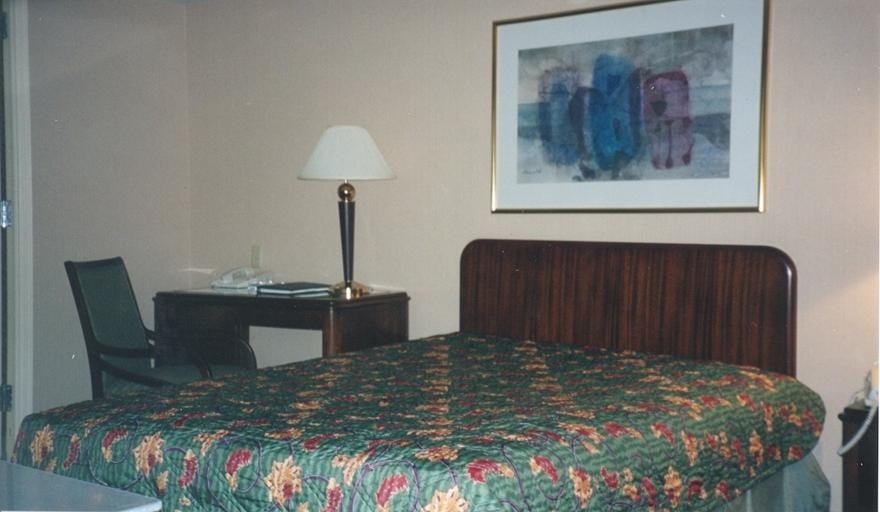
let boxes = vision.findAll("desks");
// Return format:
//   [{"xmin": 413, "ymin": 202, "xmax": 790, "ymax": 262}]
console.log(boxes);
[{"xmin": 151, "ymin": 289, "xmax": 412, "ymax": 363}]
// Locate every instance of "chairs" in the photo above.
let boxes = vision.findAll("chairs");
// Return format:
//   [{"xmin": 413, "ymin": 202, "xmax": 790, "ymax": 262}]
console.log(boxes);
[{"xmin": 62, "ymin": 257, "xmax": 259, "ymax": 400}]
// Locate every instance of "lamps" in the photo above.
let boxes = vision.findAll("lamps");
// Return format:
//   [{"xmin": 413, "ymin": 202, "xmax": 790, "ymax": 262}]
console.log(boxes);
[{"xmin": 296, "ymin": 124, "xmax": 397, "ymax": 302}]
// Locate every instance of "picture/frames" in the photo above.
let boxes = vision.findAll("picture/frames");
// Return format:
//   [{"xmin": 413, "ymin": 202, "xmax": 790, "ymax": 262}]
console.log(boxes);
[{"xmin": 491, "ymin": 1, "xmax": 772, "ymax": 214}]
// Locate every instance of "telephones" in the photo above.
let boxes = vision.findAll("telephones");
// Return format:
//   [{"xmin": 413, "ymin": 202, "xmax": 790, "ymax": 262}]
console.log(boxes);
[
  {"xmin": 864, "ymin": 364, "xmax": 878, "ymax": 407},
  {"xmin": 210, "ymin": 267, "xmax": 273, "ymax": 289}
]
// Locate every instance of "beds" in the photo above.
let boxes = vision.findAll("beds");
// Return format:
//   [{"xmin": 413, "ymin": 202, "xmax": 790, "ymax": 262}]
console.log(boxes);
[{"xmin": 11, "ymin": 238, "xmax": 798, "ymax": 511}]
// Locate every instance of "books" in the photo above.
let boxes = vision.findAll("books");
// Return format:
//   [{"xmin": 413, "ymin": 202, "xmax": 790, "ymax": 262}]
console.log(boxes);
[
  {"xmin": 256, "ymin": 281, "xmax": 332, "ymax": 296},
  {"xmin": 256, "ymin": 291, "xmax": 330, "ymax": 301}
]
